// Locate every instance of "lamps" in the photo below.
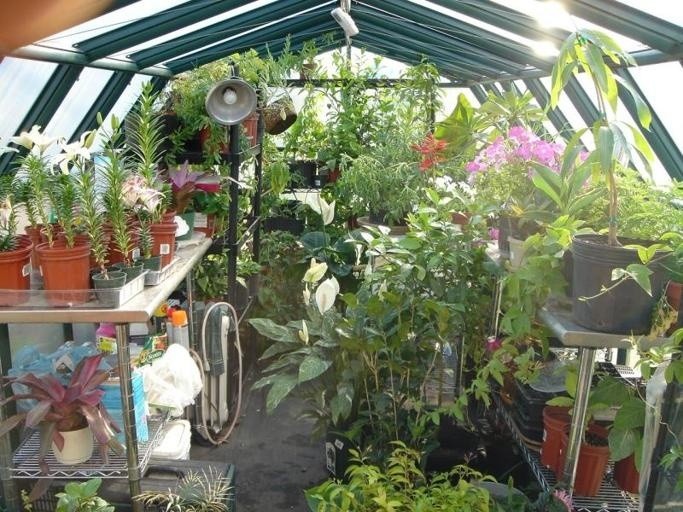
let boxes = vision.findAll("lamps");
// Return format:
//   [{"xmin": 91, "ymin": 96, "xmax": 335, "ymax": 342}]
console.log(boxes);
[{"xmin": 330, "ymin": 1, "xmax": 360, "ymax": 36}]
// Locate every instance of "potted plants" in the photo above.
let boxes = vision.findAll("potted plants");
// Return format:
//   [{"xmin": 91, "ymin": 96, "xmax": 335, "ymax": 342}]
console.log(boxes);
[{"xmin": 1, "ymin": 31, "xmax": 681, "ymax": 512}]
